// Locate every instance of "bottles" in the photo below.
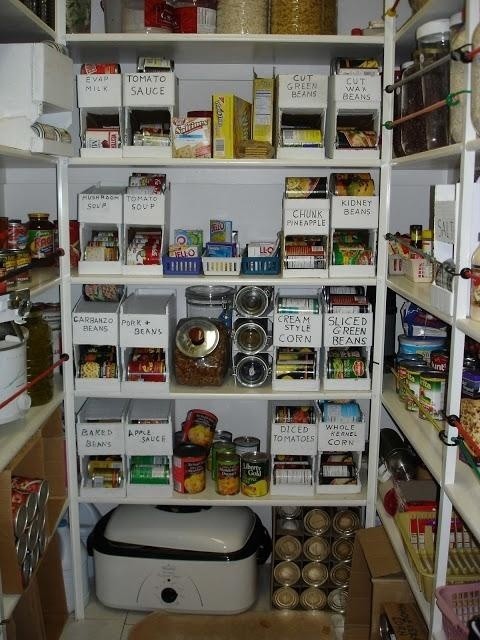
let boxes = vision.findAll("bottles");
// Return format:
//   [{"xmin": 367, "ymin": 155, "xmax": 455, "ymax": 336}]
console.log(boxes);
[
  {"xmin": 98, "ymin": 0, "xmax": 338, "ymax": 34},
  {"xmin": 394, "ymin": 11, "xmax": 480, "ymax": 158},
  {"xmin": 176, "ymin": 285, "xmax": 273, "ymax": 391},
  {"xmin": 469, "ymin": 232, "xmax": 480, "ymax": 323},
  {"xmin": 66, "ymin": 0, "xmax": 91, "ymax": 35},
  {"xmin": 24, "ymin": 0, "xmax": 55, "ymax": 31}
]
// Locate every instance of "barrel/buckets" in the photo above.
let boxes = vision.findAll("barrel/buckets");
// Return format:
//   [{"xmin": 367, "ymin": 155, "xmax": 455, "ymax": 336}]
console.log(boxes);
[{"xmin": 56, "ymin": 519, "xmax": 91, "ymax": 614}]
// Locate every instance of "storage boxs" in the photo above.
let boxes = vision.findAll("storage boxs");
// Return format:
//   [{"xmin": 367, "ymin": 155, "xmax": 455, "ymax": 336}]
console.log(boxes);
[
  {"xmin": 14, "ymin": 534, "xmax": 70, "ymax": 639},
  {"xmin": 381, "ymin": 600, "xmax": 428, "ymax": 640},
  {"xmin": 346, "ymin": 526, "xmax": 415, "ymax": 640},
  {"xmin": 36, "ymin": 409, "xmax": 67, "ymax": 534},
  {"xmin": 1, "ymin": 435, "xmax": 51, "ymax": 596},
  {"xmin": 3, "ymin": 44, "xmax": 76, "ymax": 154}
]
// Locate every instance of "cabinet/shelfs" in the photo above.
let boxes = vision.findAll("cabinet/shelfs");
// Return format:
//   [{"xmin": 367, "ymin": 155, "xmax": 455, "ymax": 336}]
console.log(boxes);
[
  {"xmin": 1, "ymin": 1, "xmax": 81, "ymax": 640},
  {"xmin": 368, "ymin": 1, "xmax": 480, "ymax": 640},
  {"xmin": 56, "ymin": 3, "xmax": 393, "ymax": 639}
]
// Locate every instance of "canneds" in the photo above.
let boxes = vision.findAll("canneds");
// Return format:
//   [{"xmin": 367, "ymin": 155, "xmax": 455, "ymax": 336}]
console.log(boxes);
[
  {"xmin": 0, "ymin": 213, "xmax": 80, "ymax": 295},
  {"xmin": 395, "ymin": 335, "xmax": 451, "ymax": 421},
  {"xmin": 280, "ymin": 56, "xmax": 383, "ymax": 151},
  {"xmin": 80, "ymin": 55, "xmax": 175, "ymax": 149},
  {"xmin": 272, "ymin": 506, "xmax": 361, "ymax": 615},
  {"xmin": 85, "ymin": 401, "xmax": 364, "ymax": 498},
  {"xmin": 77, "ymin": 285, "xmax": 369, "ymax": 389},
  {"xmin": 284, "ymin": 173, "xmax": 376, "ymax": 269},
  {"xmin": 85, "ymin": 172, "xmax": 167, "ymax": 267},
  {"xmin": 31, "ymin": 121, "xmax": 73, "ymax": 146},
  {"xmin": 11, "ymin": 475, "xmax": 50, "ymax": 587}
]
